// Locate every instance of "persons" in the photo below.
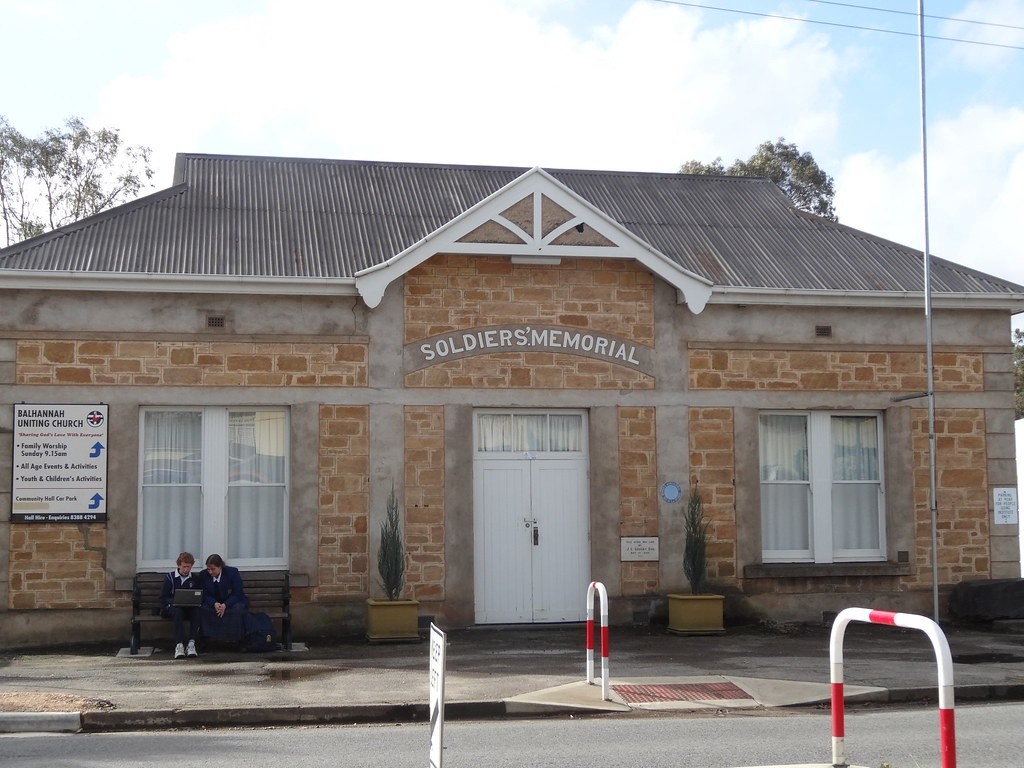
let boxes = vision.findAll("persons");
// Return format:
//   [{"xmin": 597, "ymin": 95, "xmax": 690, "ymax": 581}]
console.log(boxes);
[
  {"xmin": 191, "ymin": 554, "xmax": 250, "ymax": 653},
  {"xmin": 162, "ymin": 551, "xmax": 199, "ymax": 660}
]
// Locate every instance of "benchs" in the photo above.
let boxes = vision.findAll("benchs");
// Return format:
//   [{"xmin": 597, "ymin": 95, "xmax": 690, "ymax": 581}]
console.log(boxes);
[{"xmin": 130, "ymin": 570, "xmax": 292, "ymax": 655}]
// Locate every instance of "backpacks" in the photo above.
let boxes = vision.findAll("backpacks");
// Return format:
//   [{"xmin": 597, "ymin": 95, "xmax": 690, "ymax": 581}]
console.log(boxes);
[{"xmin": 244, "ymin": 612, "xmax": 277, "ymax": 652}]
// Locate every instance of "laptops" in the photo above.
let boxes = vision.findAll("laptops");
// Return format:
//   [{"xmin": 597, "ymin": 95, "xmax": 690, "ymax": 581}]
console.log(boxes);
[{"xmin": 172, "ymin": 589, "xmax": 203, "ymax": 607}]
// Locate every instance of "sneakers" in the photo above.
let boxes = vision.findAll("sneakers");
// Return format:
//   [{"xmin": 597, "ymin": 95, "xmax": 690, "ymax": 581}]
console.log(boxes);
[
  {"xmin": 175, "ymin": 643, "xmax": 185, "ymax": 658},
  {"xmin": 186, "ymin": 641, "xmax": 198, "ymax": 657}
]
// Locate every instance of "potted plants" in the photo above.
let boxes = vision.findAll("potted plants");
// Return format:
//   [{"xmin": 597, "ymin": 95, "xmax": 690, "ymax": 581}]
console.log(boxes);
[
  {"xmin": 667, "ymin": 482, "xmax": 725, "ymax": 636},
  {"xmin": 365, "ymin": 482, "xmax": 420, "ymax": 642}
]
